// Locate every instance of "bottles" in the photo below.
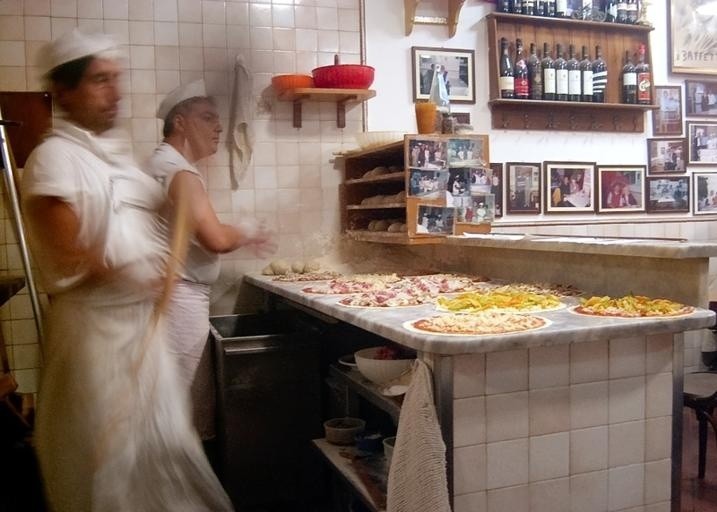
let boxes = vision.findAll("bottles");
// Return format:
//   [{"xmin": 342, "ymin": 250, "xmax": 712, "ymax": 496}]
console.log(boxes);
[
  {"xmin": 496, "ymin": 1, "xmax": 652, "ymax": 106},
  {"xmin": 427, "ymin": 65, "xmax": 450, "ymax": 133},
  {"xmin": 441, "ymin": 113, "xmax": 454, "ymax": 133}
]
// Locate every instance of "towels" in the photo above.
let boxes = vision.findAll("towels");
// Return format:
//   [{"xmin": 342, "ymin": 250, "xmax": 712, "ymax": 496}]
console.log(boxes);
[{"xmin": 387, "ymin": 358, "xmax": 453, "ymax": 512}]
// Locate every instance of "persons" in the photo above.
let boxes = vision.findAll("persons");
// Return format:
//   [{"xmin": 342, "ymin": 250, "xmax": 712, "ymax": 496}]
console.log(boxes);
[
  {"xmin": 656, "ymin": 91, "xmax": 668, "ymax": 133},
  {"xmin": 440, "ymin": 65, "xmax": 450, "ymax": 94},
  {"xmin": 606, "ymin": 180, "xmax": 631, "ymax": 207},
  {"xmin": 424, "ymin": 64, "xmax": 434, "ymax": 93},
  {"xmin": 19, "ymin": 27, "xmax": 234, "ymax": 512},
  {"xmin": 410, "ymin": 142, "xmax": 492, "ymax": 232},
  {"xmin": 139, "ymin": 79, "xmax": 277, "ymax": 443}
]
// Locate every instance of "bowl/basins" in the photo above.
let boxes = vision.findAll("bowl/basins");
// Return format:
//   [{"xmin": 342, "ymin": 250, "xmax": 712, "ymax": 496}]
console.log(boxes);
[
  {"xmin": 271, "ymin": 75, "xmax": 315, "ymax": 93},
  {"xmin": 356, "ymin": 347, "xmax": 414, "ymax": 386},
  {"xmin": 312, "ymin": 65, "xmax": 373, "ymax": 90}
]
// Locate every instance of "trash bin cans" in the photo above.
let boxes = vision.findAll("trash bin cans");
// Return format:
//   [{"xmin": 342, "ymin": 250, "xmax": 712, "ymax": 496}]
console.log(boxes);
[{"xmin": 209, "ymin": 308, "xmax": 328, "ymax": 512}]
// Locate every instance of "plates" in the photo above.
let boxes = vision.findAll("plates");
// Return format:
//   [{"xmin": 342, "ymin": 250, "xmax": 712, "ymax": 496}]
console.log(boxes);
[{"xmin": 337, "ymin": 355, "xmax": 359, "ymax": 373}]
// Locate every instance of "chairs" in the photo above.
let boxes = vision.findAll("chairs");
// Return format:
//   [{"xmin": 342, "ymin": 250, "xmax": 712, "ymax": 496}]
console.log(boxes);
[{"xmin": 682, "ymin": 301, "xmax": 717, "ymax": 479}]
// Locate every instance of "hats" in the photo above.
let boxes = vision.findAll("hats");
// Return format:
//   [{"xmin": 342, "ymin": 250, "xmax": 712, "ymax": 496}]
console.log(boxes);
[
  {"xmin": 36, "ymin": 27, "xmax": 111, "ymax": 76},
  {"xmin": 155, "ymin": 78, "xmax": 207, "ymax": 119}
]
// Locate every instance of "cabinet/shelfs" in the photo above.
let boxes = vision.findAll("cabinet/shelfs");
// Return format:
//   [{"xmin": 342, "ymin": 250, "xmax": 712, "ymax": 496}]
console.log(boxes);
[
  {"xmin": 338, "ymin": 136, "xmax": 490, "ymax": 244},
  {"xmin": 312, "ymin": 366, "xmax": 404, "ymax": 512},
  {"xmin": 487, "ymin": 12, "xmax": 661, "ymax": 110}
]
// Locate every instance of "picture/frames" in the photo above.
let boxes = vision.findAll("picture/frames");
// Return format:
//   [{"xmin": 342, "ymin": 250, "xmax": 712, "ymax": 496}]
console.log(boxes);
[
  {"xmin": 490, "ymin": 78, "xmax": 717, "ymax": 218},
  {"xmin": 412, "ymin": 46, "xmax": 476, "ymax": 104},
  {"xmin": 666, "ymin": 0, "xmax": 717, "ymax": 78}
]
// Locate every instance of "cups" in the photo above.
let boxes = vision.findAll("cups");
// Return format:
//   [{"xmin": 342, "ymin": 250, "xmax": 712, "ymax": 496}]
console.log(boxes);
[
  {"xmin": 381, "ymin": 435, "xmax": 395, "ymax": 456},
  {"xmin": 414, "ymin": 103, "xmax": 437, "ymax": 135},
  {"xmin": 324, "ymin": 417, "xmax": 365, "ymax": 445}
]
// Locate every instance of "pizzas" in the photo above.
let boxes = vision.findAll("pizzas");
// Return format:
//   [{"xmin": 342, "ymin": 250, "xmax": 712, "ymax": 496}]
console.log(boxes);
[
  {"xmin": 414, "ymin": 311, "xmax": 546, "ymax": 335},
  {"xmin": 573, "ymin": 291, "xmax": 693, "ymax": 317}
]
